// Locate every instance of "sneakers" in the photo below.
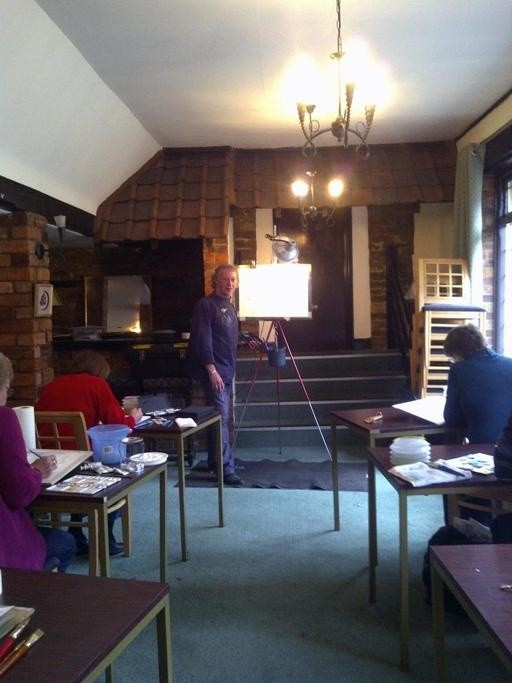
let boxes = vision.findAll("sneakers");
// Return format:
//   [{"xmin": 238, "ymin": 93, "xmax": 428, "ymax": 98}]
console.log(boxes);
[
  {"xmin": 75, "ymin": 538, "xmax": 89, "ymax": 556},
  {"xmin": 216, "ymin": 474, "xmax": 244, "ymax": 485},
  {"xmin": 209, "ymin": 462, "xmax": 245, "ymax": 472},
  {"xmin": 109, "ymin": 540, "xmax": 124, "ymax": 554}
]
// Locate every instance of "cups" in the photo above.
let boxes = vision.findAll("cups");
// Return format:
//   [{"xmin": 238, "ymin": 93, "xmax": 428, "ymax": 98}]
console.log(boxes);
[{"xmin": 181, "ymin": 332, "xmax": 190, "ymax": 340}]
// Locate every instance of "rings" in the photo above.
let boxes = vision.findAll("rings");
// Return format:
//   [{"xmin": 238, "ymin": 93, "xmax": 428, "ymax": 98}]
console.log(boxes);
[{"xmin": 53, "ymin": 459, "xmax": 56, "ymax": 463}]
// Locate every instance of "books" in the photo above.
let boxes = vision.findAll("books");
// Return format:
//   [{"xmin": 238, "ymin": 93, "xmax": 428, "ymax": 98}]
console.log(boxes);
[
  {"xmin": 121, "ymin": 392, "xmax": 216, "ymax": 430},
  {"xmin": 391, "ymin": 394, "xmax": 447, "ymax": 426},
  {"xmin": 24, "ymin": 446, "xmax": 94, "ymax": 486},
  {"xmin": 444, "ymin": 451, "xmax": 497, "ymax": 474},
  {"xmin": 1, "ymin": 604, "xmax": 38, "ymax": 639}
]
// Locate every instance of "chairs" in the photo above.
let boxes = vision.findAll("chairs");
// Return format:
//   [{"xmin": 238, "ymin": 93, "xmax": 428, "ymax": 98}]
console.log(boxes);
[
  {"xmin": 409, "ymin": 256, "xmax": 487, "ymax": 400},
  {"xmin": 34, "ymin": 411, "xmax": 130, "ymax": 574}
]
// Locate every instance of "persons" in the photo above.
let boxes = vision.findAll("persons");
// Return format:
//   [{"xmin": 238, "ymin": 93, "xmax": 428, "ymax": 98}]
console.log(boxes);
[
  {"xmin": 488, "ymin": 413, "xmax": 512, "ymax": 542},
  {"xmin": 438, "ymin": 323, "xmax": 511, "ymax": 526},
  {"xmin": 1, "ymin": 353, "xmax": 77, "ymax": 574},
  {"xmin": 34, "ymin": 350, "xmax": 145, "ymax": 559},
  {"xmin": 187, "ymin": 262, "xmax": 246, "ymax": 486}
]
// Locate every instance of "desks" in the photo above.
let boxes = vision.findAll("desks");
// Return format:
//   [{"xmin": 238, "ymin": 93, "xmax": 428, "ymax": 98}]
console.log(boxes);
[
  {"xmin": 31, "ymin": 461, "xmax": 169, "ymax": 583},
  {"xmin": 132, "ymin": 412, "xmax": 224, "ymax": 562},
  {"xmin": 333, "ymin": 393, "xmax": 512, "ymax": 682},
  {"xmin": 0, "ymin": 570, "xmax": 173, "ymax": 683}
]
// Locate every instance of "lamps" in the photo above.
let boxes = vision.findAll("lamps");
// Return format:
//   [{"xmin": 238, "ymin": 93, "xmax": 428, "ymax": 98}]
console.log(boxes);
[
  {"xmin": 37, "ymin": 213, "xmax": 66, "ymax": 258},
  {"xmin": 297, "ymin": 0, "xmax": 374, "ymax": 161},
  {"xmin": 291, "ymin": 166, "xmax": 344, "ymax": 227}
]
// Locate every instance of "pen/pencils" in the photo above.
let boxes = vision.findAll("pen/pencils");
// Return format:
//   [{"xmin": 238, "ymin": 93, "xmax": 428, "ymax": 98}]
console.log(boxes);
[{"xmin": 163, "ymin": 420, "xmax": 174, "ymax": 427}]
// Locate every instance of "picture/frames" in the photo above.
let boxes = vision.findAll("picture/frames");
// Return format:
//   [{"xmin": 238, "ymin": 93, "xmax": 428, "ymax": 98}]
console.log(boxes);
[{"xmin": 34, "ymin": 282, "xmax": 53, "ymax": 319}]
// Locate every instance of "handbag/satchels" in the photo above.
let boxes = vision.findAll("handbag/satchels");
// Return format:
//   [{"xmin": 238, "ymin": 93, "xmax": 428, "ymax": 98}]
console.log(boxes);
[{"xmin": 424, "ymin": 527, "xmax": 469, "ymax": 610}]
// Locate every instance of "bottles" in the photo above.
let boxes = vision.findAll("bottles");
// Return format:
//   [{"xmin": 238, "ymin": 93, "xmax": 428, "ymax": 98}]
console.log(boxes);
[{"xmin": 118, "ymin": 438, "xmax": 145, "ymax": 477}]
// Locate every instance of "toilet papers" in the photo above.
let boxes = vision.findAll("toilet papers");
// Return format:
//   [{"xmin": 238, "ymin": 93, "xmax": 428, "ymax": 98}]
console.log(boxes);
[{"xmin": 12, "ymin": 406, "xmax": 36, "ymax": 450}]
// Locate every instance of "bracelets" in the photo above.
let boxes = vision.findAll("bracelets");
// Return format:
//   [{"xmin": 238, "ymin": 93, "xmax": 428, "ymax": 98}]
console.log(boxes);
[{"xmin": 208, "ymin": 368, "xmax": 217, "ymax": 375}]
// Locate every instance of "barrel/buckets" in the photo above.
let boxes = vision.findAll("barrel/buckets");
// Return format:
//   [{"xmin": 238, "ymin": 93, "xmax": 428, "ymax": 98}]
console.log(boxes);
[
  {"xmin": 267, "ymin": 335, "xmax": 286, "ymax": 367},
  {"xmin": 85, "ymin": 424, "xmax": 132, "ymax": 464}
]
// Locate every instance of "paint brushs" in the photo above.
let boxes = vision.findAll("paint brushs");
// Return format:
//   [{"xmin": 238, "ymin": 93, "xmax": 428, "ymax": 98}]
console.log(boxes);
[
  {"xmin": 30, "ymin": 449, "xmax": 42, "ymax": 459},
  {"xmin": 0, "ymin": 617, "xmax": 44, "ymax": 678},
  {"xmin": 427, "ymin": 462, "xmax": 465, "ymax": 478}
]
